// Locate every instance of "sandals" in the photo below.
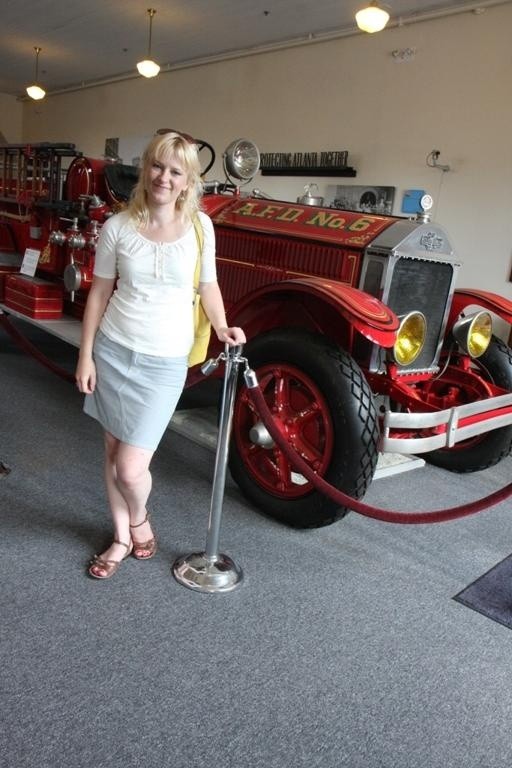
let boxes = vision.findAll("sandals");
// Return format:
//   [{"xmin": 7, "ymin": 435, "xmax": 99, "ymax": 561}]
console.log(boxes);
[
  {"xmin": 129, "ymin": 513, "xmax": 157, "ymax": 561},
  {"xmin": 88, "ymin": 536, "xmax": 133, "ymax": 579}
]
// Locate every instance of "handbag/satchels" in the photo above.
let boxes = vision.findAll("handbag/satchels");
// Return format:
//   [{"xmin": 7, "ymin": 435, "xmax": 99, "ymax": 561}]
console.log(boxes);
[{"xmin": 187, "ymin": 213, "xmax": 212, "ymax": 368}]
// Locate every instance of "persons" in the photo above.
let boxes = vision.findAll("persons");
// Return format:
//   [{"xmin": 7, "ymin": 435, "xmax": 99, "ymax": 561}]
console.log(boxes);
[{"xmin": 74, "ymin": 127, "xmax": 249, "ymax": 582}]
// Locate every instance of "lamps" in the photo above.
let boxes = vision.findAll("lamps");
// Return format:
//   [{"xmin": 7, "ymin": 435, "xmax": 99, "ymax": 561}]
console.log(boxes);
[
  {"xmin": 25, "ymin": 47, "xmax": 46, "ymax": 101},
  {"xmin": 354, "ymin": 0, "xmax": 390, "ymax": 33},
  {"xmin": 136, "ymin": 8, "xmax": 161, "ymax": 78}
]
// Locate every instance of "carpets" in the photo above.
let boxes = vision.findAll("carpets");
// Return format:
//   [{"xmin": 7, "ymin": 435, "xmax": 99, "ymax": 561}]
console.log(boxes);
[{"xmin": 451, "ymin": 552, "xmax": 512, "ymax": 630}]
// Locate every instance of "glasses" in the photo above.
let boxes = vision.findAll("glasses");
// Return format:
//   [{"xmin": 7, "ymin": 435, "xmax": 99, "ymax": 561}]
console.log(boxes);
[{"xmin": 157, "ymin": 128, "xmax": 196, "ymax": 144}]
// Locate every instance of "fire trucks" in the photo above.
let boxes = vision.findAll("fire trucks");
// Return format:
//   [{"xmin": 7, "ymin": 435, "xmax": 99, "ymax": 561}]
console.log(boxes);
[{"xmin": 0, "ymin": 136, "xmax": 512, "ymax": 529}]
